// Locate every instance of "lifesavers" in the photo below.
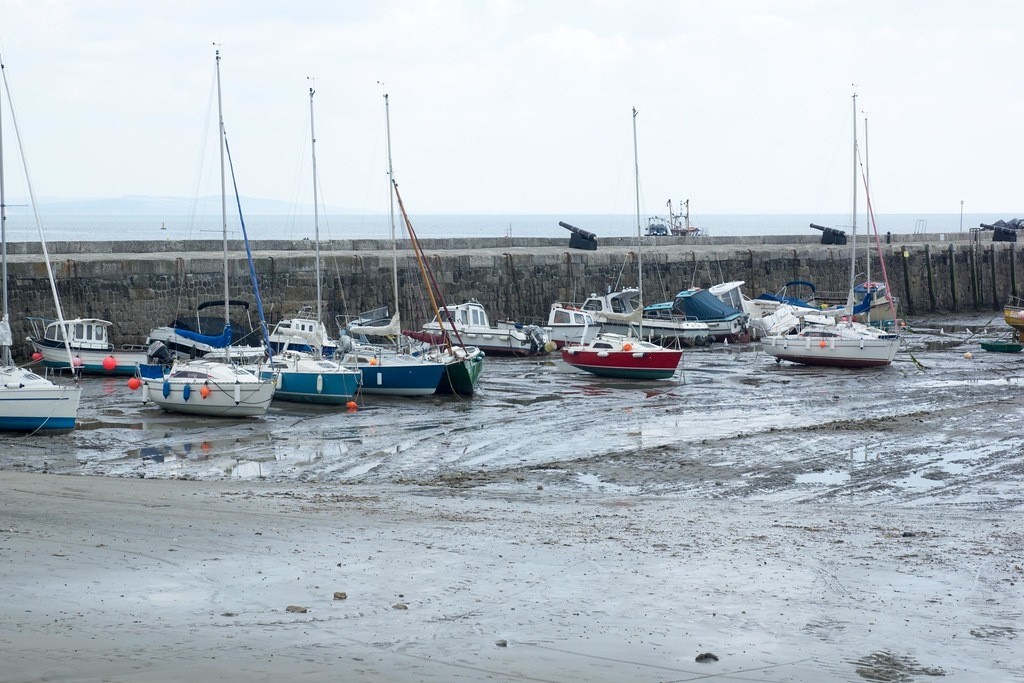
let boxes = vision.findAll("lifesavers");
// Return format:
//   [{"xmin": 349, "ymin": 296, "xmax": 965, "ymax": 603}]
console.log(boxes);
[
  {"xmin": 864, "ymin": 282, "xmax": 878, "ymax": 288},
  {"xmin": 688, "ymin": 288, "xmax": 700, "ymax": 290},
  {"xmin": 566, "ymin": 307, "xmax": 580, "ymax": 311}
]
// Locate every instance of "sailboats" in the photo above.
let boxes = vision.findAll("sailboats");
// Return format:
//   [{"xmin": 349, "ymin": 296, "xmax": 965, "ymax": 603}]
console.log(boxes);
[
  {"xmin": 376, "ymin": 89, "xmax": 484, "ymax": 397},
  {"xmin": 301, "ymin": 85, "xmax": 449, "ymax": 397},
  {"xmin": 136, "ymin": 41, "xmax": 282, "ymax": 417},
  {"xmin": 562, "ymin": 105, "xmax": 685, "ymax": 380},
  {"xmin": 0, "ymin": 43, "xmax": 85, "ymax": 434},
  {"xmin": 205, "ymin": 75, "xmax": 364, "ymax": 407},
  {"xmin": 760, "ymin": 83, "xmax": 902, "ymax": 367}
]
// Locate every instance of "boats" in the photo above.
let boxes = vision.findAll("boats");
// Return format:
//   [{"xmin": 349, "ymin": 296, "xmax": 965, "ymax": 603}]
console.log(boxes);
[
  {"xmin": 979, "ymin": 294, "xmax": 1024, "ymax": 353},
  {"xmin": 643, "ymin": 196, "xmax": 707, "ymax": 238},
  {"xmin": 147, "ymin": 238, "xmax": 896, "ymax": 363},
  {"xmin": 19, "ymin": 314, "xmax": 170, "ymax": 379}
]
